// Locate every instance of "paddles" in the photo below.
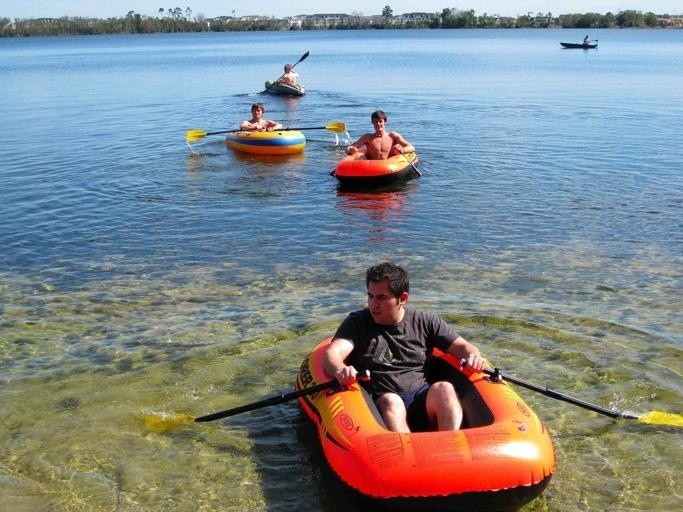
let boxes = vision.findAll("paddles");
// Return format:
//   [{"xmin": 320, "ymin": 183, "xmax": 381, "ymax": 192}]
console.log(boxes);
[
  {"xmin": 142, "ymin": 370, "xmax": 372, "ymax": 433},
  {"xmin": 461, "ymin": 359, "xmax": 683, "ymax": 428},
  {"xmin": 186, "ymin": 127, "xmax": 265, "ymax": 142},
  {"xmin": 576, "ymin": 40, "xmax": 598, "ymax": 44},
  {"xmin": 266, "ymin": 121, "xmax": 345, "ymax": 132},
  {"xmin": 263, "ymin": 51, "xmax": 310, "ymax": 94}
]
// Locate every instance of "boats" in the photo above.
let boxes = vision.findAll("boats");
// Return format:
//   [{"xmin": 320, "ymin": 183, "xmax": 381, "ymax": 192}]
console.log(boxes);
[
  {"xmin": 333, "ymin": 141, "xmax": 421, "ymax": 193},
  {"xmin": 263, "ymin": 80, "xmax": 303, "ymax": 97},
  {"xmin": 292, "ymin": 334, "xmax": 555, "ymax": 511},
  {"xmin": 561, "ymin": 39, "xmax": 599, "ymax": 53},
  {"xmin": 225, "ymin": 125, "xmax": 306, "ymax": 156}
]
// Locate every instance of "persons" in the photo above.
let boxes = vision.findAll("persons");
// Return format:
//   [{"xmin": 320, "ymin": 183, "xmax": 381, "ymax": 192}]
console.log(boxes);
[
  {"xmin": 583, "ymin": 34, "xmax": 591, "ymax": 48},
  {"xmin": 325, "ymin": 263, "xmax": 487, "ymax": 434},
  {"xmin": 241, "ymin": 104, "xmax": 283, "ymax": 132},
  {"xmin": 278, "ymin": 65, "xmax": 298, "ymax": 85},
  {"xmin": 346, "ymin": 111, "xmax": 415, "ymax": 159}
]
